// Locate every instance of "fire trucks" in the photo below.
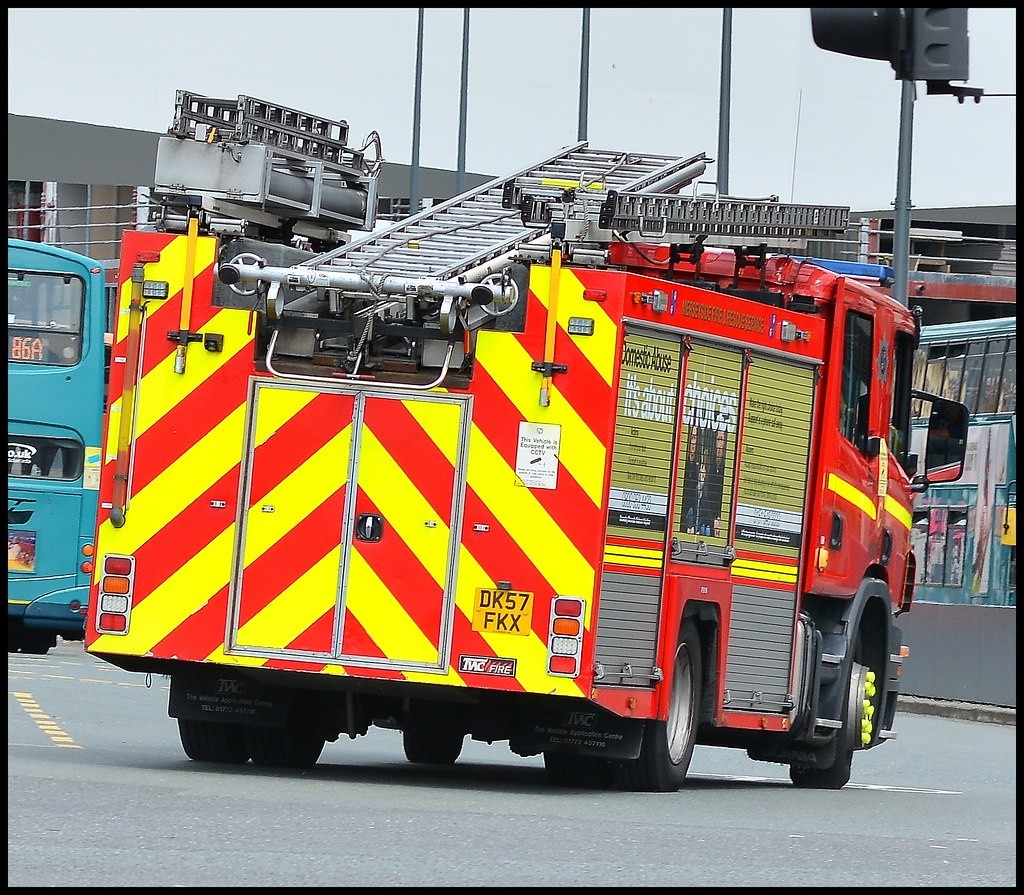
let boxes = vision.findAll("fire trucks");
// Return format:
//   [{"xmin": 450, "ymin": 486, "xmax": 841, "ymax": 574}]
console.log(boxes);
[{"xmin": 83, "ymin": 89, "xmax": 971, "ymax": 794}]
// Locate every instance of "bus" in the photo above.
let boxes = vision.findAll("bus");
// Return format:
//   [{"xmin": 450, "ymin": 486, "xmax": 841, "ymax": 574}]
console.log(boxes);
[
  {"xmin": 909, "ymin": 315, "xmax": 1017, "ymax": 608},
  {"xmin": 6, "ymin": 236, "xmax": 107, "ymax": 655}
]
